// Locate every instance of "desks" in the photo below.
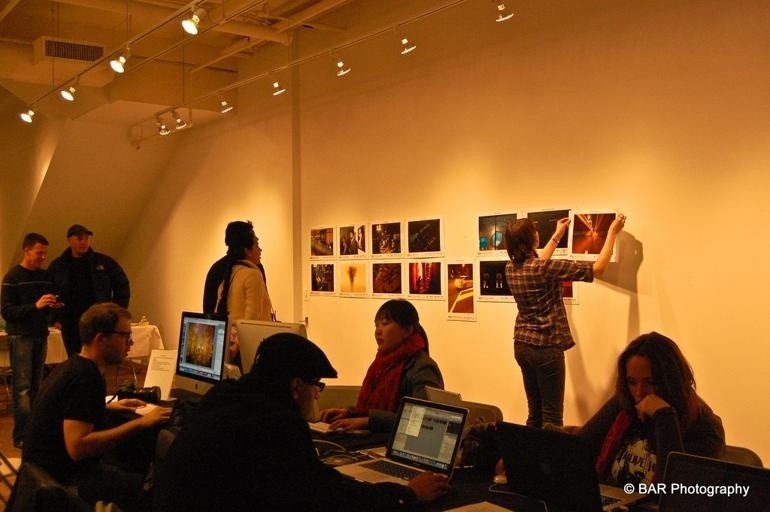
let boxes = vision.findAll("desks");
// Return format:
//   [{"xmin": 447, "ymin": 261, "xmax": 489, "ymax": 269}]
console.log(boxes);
[
  {"xmin": 0, "ymin": 317, "xmax": 164, "ymax": 389},
  {"xmin": 137, "ymin": 389, "xmax": 516, "ymax": 511}
]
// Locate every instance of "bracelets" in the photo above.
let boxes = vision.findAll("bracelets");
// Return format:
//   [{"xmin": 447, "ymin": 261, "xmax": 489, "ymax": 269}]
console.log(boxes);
[{"xmin": 551, "ymin": 236, "xmax": 560, "ymax": 245}]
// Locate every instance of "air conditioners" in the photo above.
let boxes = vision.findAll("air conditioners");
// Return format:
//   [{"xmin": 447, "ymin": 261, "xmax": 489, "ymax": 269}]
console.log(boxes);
[{"xmin": 32, "ymin": 34, "xmax": 108, "ymax": 68}]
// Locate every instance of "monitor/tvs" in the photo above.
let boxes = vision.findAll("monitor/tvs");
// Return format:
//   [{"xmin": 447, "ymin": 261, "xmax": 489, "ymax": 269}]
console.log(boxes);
[
  {"xmin": 236, "ymin": 317, "xmax": 308, "ymax": 378},
  {"xmin": 175, "ymin": 312, "xmax": 229, "ymax": 385},
  {"xmin": 423, "ymin": 385, "xmax": 504, "ymax": 425}
]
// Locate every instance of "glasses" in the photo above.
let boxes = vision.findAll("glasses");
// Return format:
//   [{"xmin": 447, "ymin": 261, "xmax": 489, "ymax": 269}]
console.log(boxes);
[
  {"xmin": 312, "ymin": 380, "xmax": 326, "ymax": 392},
  {"xmin": 113, "ymin": 330, "xmax": 132, "ymax": 341}
]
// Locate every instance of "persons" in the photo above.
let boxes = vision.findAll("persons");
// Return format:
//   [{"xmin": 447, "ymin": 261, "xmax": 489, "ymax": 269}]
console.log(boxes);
[
  {"xmin": 215, "ymin": 235, "xmax": 273, "ymax": 375},
  {"xmin": 204, "ymin": 220, "xmax": 266, "ymax": 319},
  {"xmin": 173, "ymin": 332, "xmax": 450, "ymax": 512},
  {"xmin": 46, "ymin": 226, "xmax": 131, "ymax": 355},
  {"xmin": 318, "ymin": 299, "xmax": 445, "ymax": 430},
  {"xmin": 505, "ymin": 213, "xmax": 626, "ymax": 429},
  {"xmin": 22, "ymin": 304, "xmax": 173, "ymax": 502},
  {"xmin": 573, "ymin": 332, "xmax": 726, "ymax": 493},
  {"xmin": 0, "ymin": 233, "xmax": 65, "ymax": 449},
  {"xmin": 349, "ymin": 231, "xmax": 358, "ymax": 254},
  {"xmin": 342, "ymin": 235, "xmax": 348, "ymax": 254}
]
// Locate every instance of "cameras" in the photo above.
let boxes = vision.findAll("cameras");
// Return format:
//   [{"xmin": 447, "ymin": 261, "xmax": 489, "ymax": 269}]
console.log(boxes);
[{"xmin": 117, "ymin": 378, "xmax": 162, "ymax": 404}]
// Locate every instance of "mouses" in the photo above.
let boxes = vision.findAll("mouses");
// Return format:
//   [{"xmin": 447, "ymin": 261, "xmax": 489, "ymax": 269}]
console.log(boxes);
[{"xmin": 494, "ymin": 474, "xmax": 507, "ymax": 485}]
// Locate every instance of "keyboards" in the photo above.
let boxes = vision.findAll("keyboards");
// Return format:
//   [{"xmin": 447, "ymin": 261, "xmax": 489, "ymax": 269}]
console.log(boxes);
[
  {"xmin": 309, "ymin": 421, "xmax": 341, "ymax": 432},
  {"xmin": 134, "ymin": 403, "xmax": 159, "ymax": 415}
]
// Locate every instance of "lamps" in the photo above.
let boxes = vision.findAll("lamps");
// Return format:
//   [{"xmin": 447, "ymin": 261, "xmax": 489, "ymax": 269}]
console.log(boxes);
[
  {"xmin": 106, "ymin": 49, "xmax": 133, "ymax": 77},
  {"xmin": 495, "ymin": 4, "xmax": 514, "ymax": 24},
  {"xmin": 20, "ymin": 107, "xmax": 38, "ymax": 123},
  {"xmin": 62, "ymin": 80, "xmax": 80, "ymax": 103},
  {"xmin": 181, "ymin": 7, "xmax": 207, "ymax": 36},
  {"xmin": 399, "ymin": 37, "xmax": 418, "ymax": 57},
  {"xmin": 169, "ymin": 109, "xmax": 190, "ymax": 133},
  {"xmin": 265, "ymin": 78, "xmax": 288, "ymax": 98},
  {"xmin": 218, "ymin": 96, "xmax": 236, "ymax": 116},
  {"xmin": 152, "ymin": 116, "xmax": 171, "ymax": 139},
  {"xmin": 336, "ymin": 60, "xmax": 352, "ymax": 78}
]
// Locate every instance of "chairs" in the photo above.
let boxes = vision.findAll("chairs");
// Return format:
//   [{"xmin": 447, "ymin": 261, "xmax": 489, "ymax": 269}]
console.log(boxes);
[{"xmin": 1, "ymin": 445, "xmax": 120, "ymax": 512}]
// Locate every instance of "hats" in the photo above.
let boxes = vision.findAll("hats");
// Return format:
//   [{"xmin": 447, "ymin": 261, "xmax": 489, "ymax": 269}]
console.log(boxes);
[
  {"xmin": 67, "ymin": 224, "xmax": 93, "ymax": 238},
  {"xmin": 254, "ymin": 332, "xmax": 338, "ymax": 379}
]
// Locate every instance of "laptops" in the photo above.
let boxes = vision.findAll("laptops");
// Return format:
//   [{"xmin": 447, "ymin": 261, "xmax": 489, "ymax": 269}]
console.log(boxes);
[
  {"xmin": 658, "ymin": 452, "xmax": 770, "ymax": 512},
  {"xmin": 496, "ymin": 421, "xmax": 624, "ymax": 512},
  {"xmin": 335, "ymin": 396, "xmax": 470, "ymax": 487}
]
